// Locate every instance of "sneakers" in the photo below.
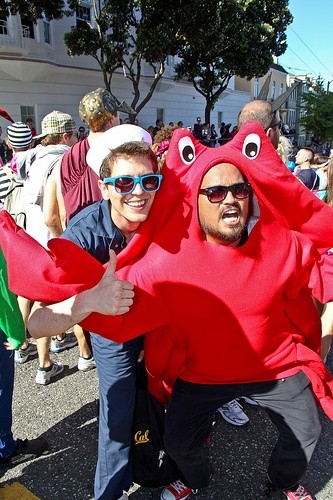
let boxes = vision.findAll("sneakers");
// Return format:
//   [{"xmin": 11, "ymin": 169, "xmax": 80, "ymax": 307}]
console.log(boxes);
[
  {"xmin": 36, "ymin": 361, "xmax": 63, "ymax": 384},
  {"xmin": 160, "ymin": 479, "xmax": 193, "ymax": 500},
  {"xmin": 14, "ymin": 343, "xmax": 37, "ymax": 363},
  {"xmin": 267, "ymin": 473, "xmax": 314, "ymax": 500},
  {"xmin": 218, "ymin": 399, "xmax": 249, "ymax": 426},
  {"xmin": 78, "ymin": 354, "xmax": 96, "ymax": 371},
  {"xmin": 50, "ymin": 333, "xmax": 78, "ymax": 353}
]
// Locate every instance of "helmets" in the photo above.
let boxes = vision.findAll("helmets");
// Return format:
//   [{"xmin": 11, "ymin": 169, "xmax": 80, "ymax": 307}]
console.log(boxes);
[
  {"xmin": 78, "ymin": 88, "xmax": 137, "ymax": 132},
  {"xmin": 238, "ymin": 72, "xmax": 299, "ymax": 133}
]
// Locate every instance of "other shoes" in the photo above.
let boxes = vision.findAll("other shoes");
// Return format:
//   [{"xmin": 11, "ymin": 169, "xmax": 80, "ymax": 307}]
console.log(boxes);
[{"xmin": 0, "ymin": 439, "xmax": 47, "ymax": 469}]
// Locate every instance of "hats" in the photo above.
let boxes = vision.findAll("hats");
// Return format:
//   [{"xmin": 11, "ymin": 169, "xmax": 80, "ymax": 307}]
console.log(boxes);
[
  {"xmin": 86, "ymin": 124, "xmax": 152, "ymax": 178},
  {"xmin": 7, "ymin": 122, "xmax": 32, "ymax": 148},
  {"xmin": 33, "ymin": 111, "xmax": 76, "ymax": 140}
]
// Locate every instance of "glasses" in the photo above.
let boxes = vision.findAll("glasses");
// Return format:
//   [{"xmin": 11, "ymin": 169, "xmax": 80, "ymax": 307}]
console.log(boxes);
[
  {"xmin": 263, "ymin": 121, "xmax": 283, "ymax": 133},
  {"xmin": 103, "ymin": 174, "xmax": 162, "ymax": 194},
  {"xmin": 198, "ymin": 182, "xmax": 251, "ymax": 204}
]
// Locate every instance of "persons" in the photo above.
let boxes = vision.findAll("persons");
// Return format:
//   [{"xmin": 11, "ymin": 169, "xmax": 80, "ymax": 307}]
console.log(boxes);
[
  {"xmin": 0, "ymin": 246, "xmax": 47, "ymax": 465},
  {"xmin": 277, "ymin": 136, "xmax": 333, "ymax": 203},
  {"xmin": 148, "ymin": 116, "xmax": 298, "ymax": 150},
  {"xmin": 0, "ymin": 88, "xmax": 165, "ymax": 384},
  {"xmin": 0, "ymin": 118, "xmax": 333, "ymax": 500},
  {"xmin": 26, "ymin": 123, "xmax": 164, "ymax": 500},
  {"xmin": 215, "ymin": 98, "xmax": 281, "ymax": 425}
]
